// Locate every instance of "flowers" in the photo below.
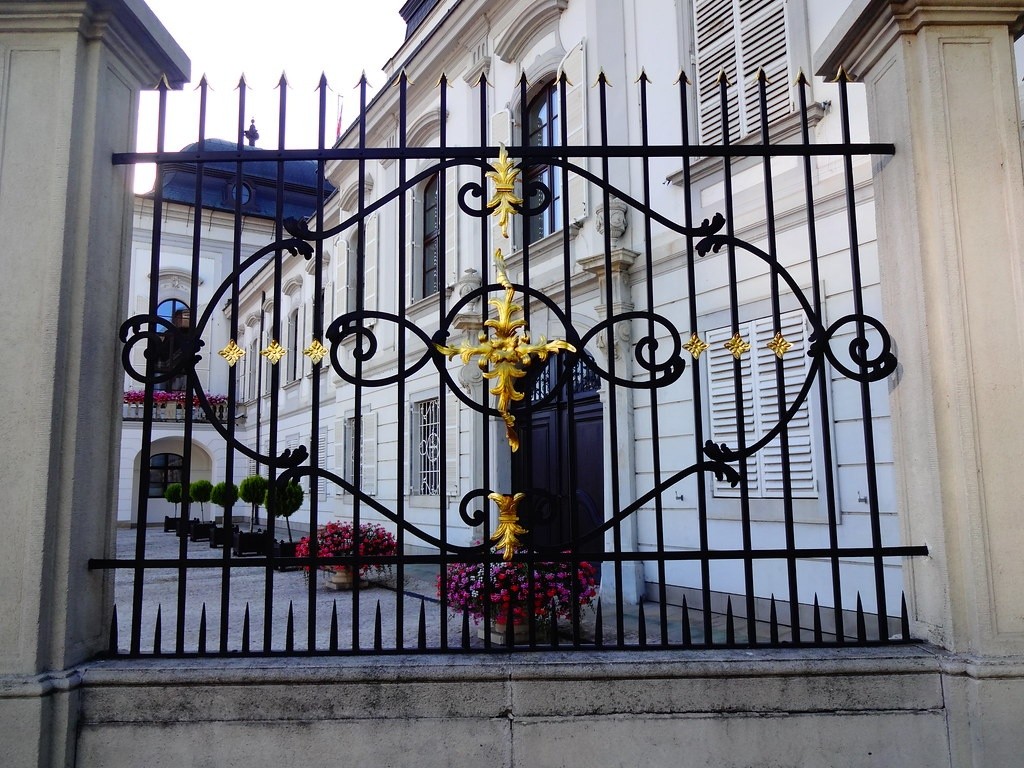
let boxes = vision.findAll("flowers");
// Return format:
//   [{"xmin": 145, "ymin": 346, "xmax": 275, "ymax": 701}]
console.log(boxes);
[
  {"xmin": 295, "ymin": 521, "xmax": 397, "ymax": 584},
  {"xmin": 437, "ymin": 537, "xmax": 596, "ymax": 626}
]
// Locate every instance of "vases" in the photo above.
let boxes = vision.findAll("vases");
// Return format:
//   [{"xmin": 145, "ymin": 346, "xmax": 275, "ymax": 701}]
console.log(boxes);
[
  {"xmin": 326, "ymin": 566, "xmax": 368, "ymax": 590},
  {"xmin": 478, "ymin": 617, "xmax": 549, "ymax": 645}
]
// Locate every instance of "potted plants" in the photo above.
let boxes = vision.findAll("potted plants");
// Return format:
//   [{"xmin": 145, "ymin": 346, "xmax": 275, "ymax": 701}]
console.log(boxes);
[
  {"xmin": 190, "ymin": 480, "xmax": 217, "ymax": 542},
  {"xmin": 264, "ymin": 481, "xmax": 306, "ymax": 573},
  {"xmin": 209, "ymin": 481, "xmax": 239, "ymax": 548},
  {"xmin": 176, "ymin": 483, "xmax": 199, "ymax": 537},
  {"xmin": 232, "ymin": 476, "xmax": 268, "ymax": 556},
  {"xmin": 164, "ymin": 483, "xmax": 182, "ymax": 532}
]
